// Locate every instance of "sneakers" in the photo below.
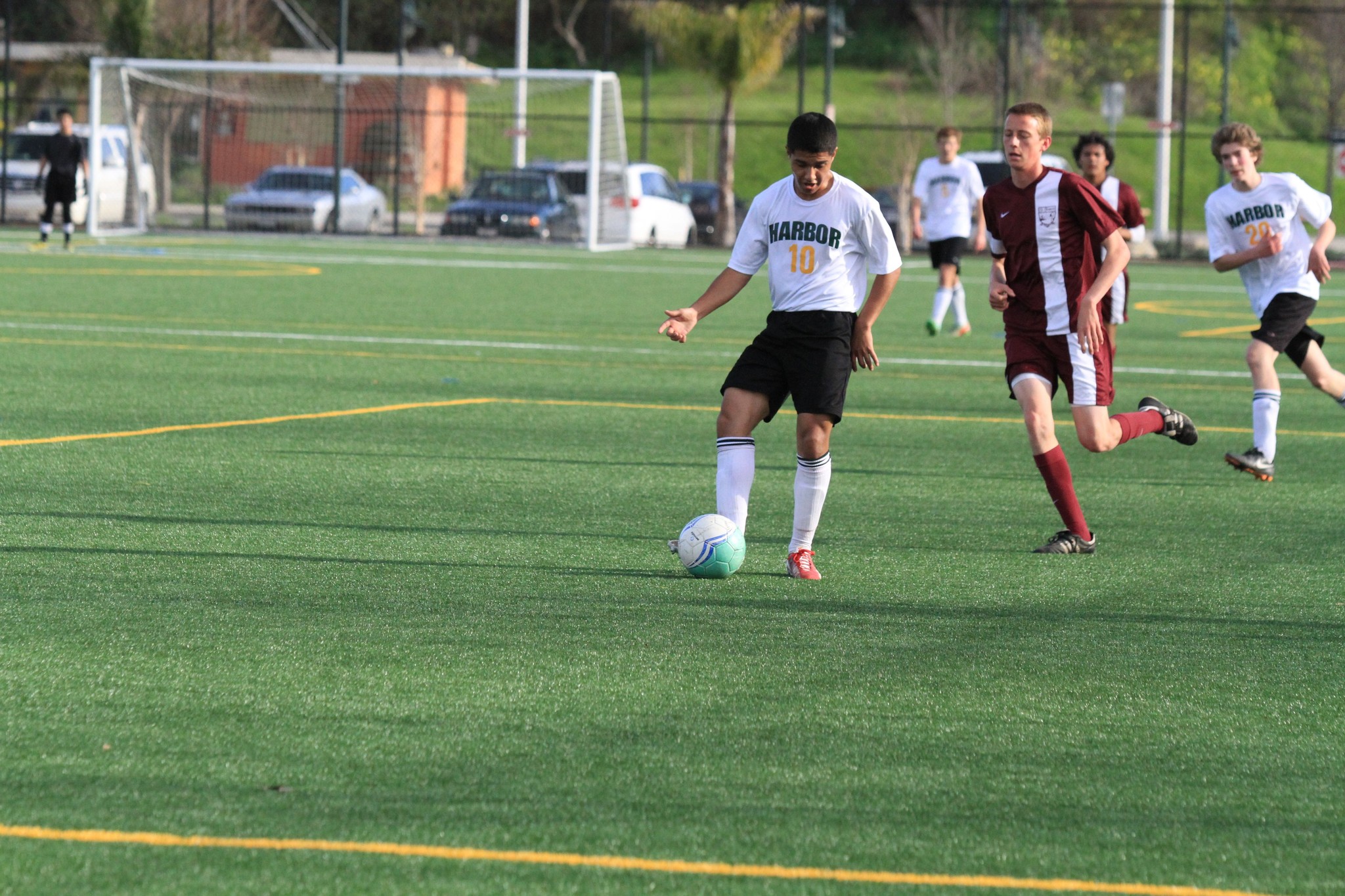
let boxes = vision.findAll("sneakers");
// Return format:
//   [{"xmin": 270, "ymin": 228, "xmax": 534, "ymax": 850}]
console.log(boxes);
[
  {"xmin": 786, "ymin": 549, "xmax": 821, "ymax": 579},
  {"xmin": 1138, "ymin": 396, "xmax": 1198, "ymax": 446},
  {"xmin": 668, "ymin": 540, "xmax": 680, "ymax": 559},
  {"xmin": 949, "ymin": 327, "xmax": 971, "ymax": 336},
  {"xmin": 1224, "ymin": 446, "xmax": 1275, "ymax": 482},
  {"xmin": 926, "ymin": 320, "xmax": 937, "ymax": 337},
  {"xmin": 1033, "ymin": 531, "xmax": 1096, "ymax": 553}
]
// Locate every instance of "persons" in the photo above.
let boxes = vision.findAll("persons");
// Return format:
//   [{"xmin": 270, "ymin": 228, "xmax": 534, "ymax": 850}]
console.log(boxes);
[
  {"xmin": 34, "ymin": 108, "xmax": 89, "ymax": 254},
  {"xmin": 982, "ymin": 103, "xmax": 1198, "ymax": 558},
  {"xmin": 909, "ymin": 127, "xmax": 987, "ymax": 340},
  {"xmin": 1204, "ymin": 121, "xmax": 1345, "ymax": 482},
  {"xmin": 1071, "ymin": 131, "xmax": 1147, "ymax": 368},
  {"xmin": 658, "ymin": 112, "xmax": 904, "ymax": 581}
]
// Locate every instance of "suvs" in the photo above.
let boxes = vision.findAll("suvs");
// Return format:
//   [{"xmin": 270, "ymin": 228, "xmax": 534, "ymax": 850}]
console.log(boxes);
[
  {"xmin": 1, "ymin": 121, "xmax": 157, "ymax": 227},
  {"xmin": 559, "ymin": 160, "xmax": 699, "ymax": 247}
]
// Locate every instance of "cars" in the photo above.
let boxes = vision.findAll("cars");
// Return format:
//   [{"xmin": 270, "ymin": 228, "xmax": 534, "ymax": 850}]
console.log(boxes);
[
  {"xmin": 223, "ymin": 165, "xmax": 394, "ymax": 237},
  {"xmin": 676, "ymin": 182, "xmax": 748, "ymax": 242},
  {"xmin": 439, "ymin": 167, "xmax": 583, "ymax": 244}
]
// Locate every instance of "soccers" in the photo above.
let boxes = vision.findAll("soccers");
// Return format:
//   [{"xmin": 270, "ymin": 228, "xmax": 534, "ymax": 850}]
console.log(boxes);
[{"xmin": 678, "ymin": 514, "xmax": 746, "ymax": 579}]
